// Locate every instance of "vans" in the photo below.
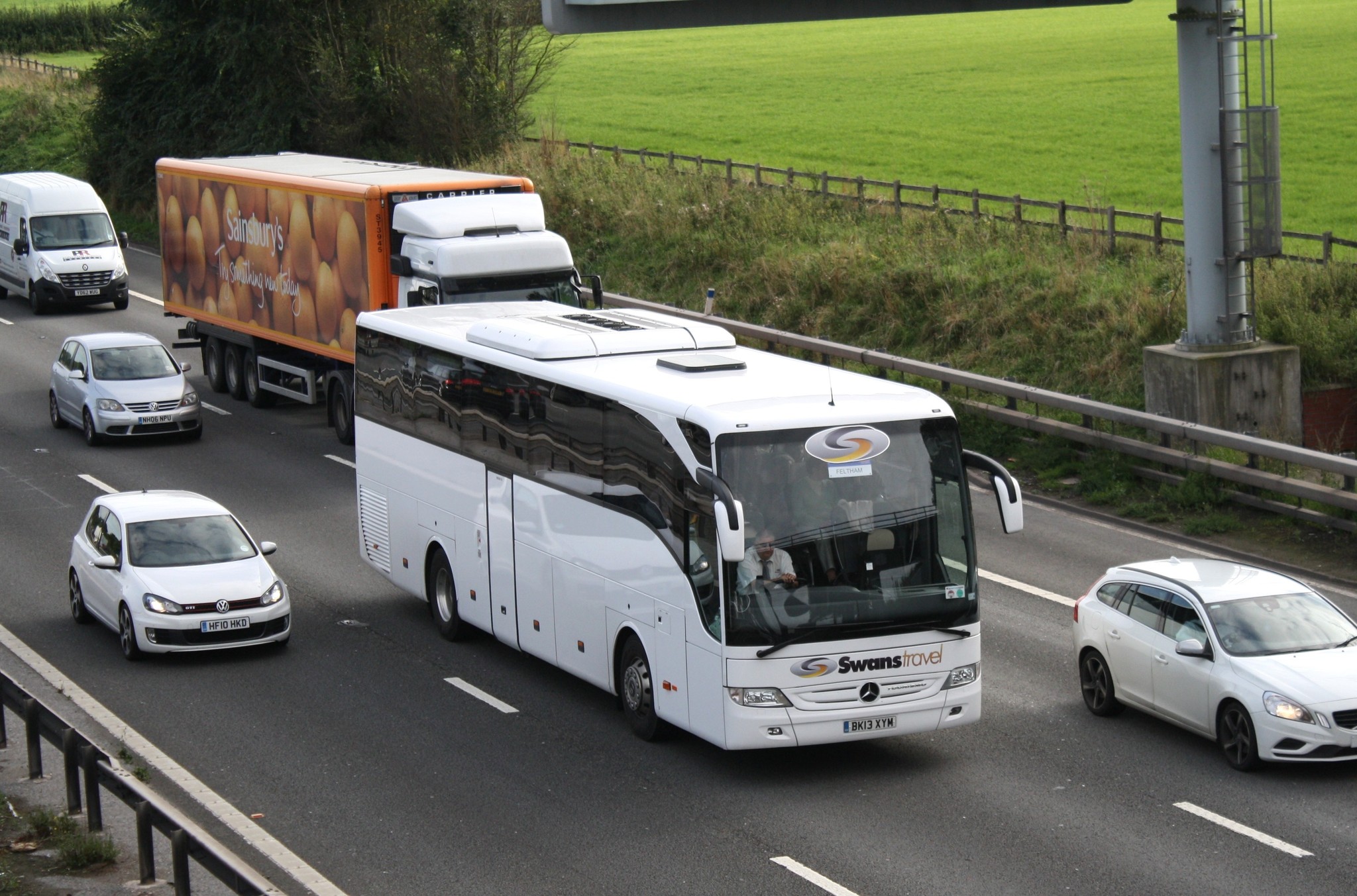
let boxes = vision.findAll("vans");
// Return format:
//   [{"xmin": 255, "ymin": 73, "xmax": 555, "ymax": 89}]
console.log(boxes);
[{"xmin": 0, "ymin": 171, "xmax": 131, "ymax": 316}]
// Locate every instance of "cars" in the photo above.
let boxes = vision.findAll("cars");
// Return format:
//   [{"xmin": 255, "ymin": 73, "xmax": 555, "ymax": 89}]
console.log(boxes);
[
  {"xmin": 67, "ymin": 488, "xmax": 293, "ymax": 661},
  {"xmin": 520, "ymin": 374, "xmax": 613, "ymax": 411},
  {"xmin": 1073, "ymin": 556, "xmax": 1357, "ymax": 773},
  {"xmin": 47, "ymin": 330, "xmax": 203, "ymax": 447},
  {"xmin": 399, "ymin": 343, "xmax": 546, "ymax": 430},
  {"xmin": 533, "ymin": 466, "xmax": 715, "ymax": 608}
]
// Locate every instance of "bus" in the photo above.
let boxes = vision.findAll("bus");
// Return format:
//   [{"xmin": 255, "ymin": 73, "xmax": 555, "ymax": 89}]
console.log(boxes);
[{"xmin": 351, "ymin": 298, "xmax": 1025, "ymax": 755}]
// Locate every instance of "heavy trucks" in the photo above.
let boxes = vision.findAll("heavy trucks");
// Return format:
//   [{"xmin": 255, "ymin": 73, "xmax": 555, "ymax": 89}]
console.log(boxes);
[{"xmin": 152, "ymin": 150, "xmax": 606, "ymax": 446}]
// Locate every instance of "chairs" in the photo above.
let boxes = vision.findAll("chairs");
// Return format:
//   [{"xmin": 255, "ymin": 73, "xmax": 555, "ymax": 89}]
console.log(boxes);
[{"xmin": 861, "ymin": 528, "xmax": 905, "ymax": 591}]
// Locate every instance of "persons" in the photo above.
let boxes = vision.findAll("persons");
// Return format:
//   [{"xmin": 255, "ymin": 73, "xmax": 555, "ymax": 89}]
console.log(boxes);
[
  {"xmin": 733, "ymin": 443, "xmax": 885, "ymax": 585},
  {"xmin": 736, "ymin": 530, "xmax": 797, "ymax": 595},
  {"xmin": 32, "ymin": 219, "xmax": 54, "ymax": 242}
]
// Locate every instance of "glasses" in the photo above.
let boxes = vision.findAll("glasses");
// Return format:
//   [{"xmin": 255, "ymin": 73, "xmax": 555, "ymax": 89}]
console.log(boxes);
[{"xmin": 761, "ymin": 543, "xmax": 775, "ymax": 547}]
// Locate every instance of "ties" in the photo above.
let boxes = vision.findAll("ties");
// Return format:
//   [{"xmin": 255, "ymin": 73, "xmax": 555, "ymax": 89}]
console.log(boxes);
[{"xmin": 761, "ymin": 561, "xmax": 770, "ymax": 580}]
